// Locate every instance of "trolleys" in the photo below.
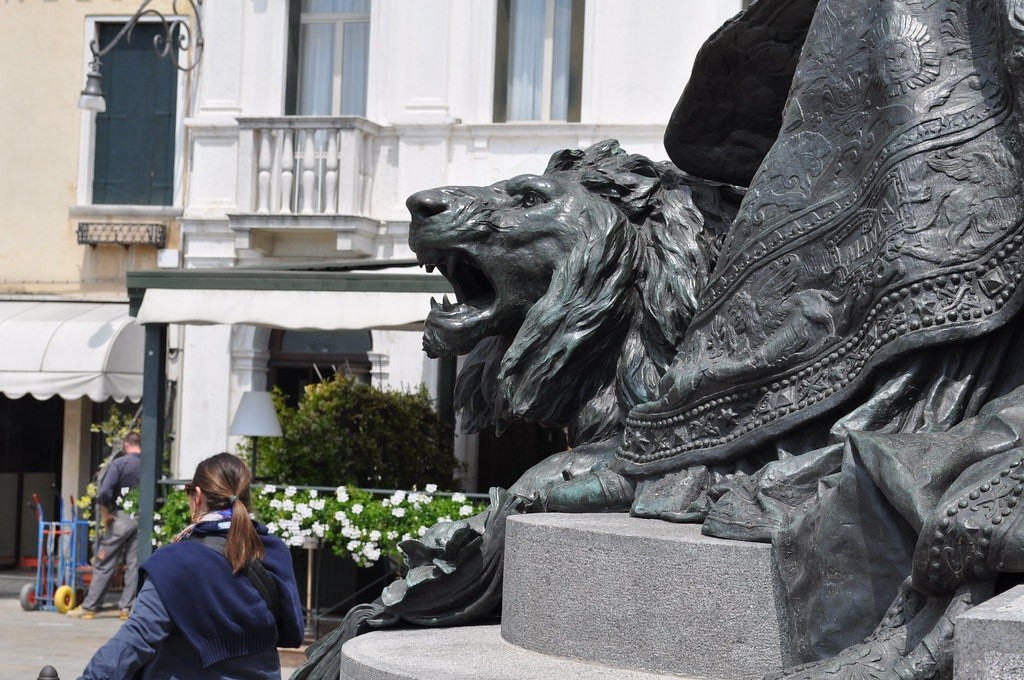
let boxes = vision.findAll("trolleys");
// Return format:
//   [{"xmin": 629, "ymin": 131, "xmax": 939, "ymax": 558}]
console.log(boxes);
[{"xmin": 17, "ymin": 492, "xmax": 90, "ymax": 612}]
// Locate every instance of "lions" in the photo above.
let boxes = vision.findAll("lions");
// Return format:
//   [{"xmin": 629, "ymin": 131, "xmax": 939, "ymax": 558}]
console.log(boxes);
[{"xmin": 404, "ymin": 137, "xmax": 1024, "ymax": 680}]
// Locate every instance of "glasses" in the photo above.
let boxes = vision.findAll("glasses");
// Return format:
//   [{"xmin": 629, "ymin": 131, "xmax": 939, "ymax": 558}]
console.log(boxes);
[{"xmin": 184, "ymin": 483, "xmax": 205, "ymax": 496}]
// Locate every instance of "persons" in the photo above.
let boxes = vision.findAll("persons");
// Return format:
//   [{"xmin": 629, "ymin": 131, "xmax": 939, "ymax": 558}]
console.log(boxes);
[
  {"xmin": 66, "ymin": 431, "xmax": 142, "ymax": 620},
  {"xmin": 77, "ymin": 452, "xmax": 304, "ymax": 680}
]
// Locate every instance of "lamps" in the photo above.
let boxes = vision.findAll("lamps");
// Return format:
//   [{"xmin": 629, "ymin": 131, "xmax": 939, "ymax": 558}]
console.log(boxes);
[{"xmin": 228, "ymin": 391, "xmax": 284, "ymax": 482}]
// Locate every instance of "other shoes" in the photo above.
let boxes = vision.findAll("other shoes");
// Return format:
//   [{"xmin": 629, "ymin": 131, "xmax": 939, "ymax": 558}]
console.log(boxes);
[
  {"xmin": 66, "ymin": 604, "xmax": 95, "ymax": 619},
  {"xmin": 120, "ymin": 608, "xmax": 130, "ymax": 619}
]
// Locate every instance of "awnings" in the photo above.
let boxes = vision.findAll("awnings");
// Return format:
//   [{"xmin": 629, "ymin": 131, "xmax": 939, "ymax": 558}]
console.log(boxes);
[
  {"xmin": 135, "ymin": 288, "xmax": 457, "ymax": 332},
  {"xmin": 0, "ymin": 300, "xmax": 146, "ymax": 404}
]
